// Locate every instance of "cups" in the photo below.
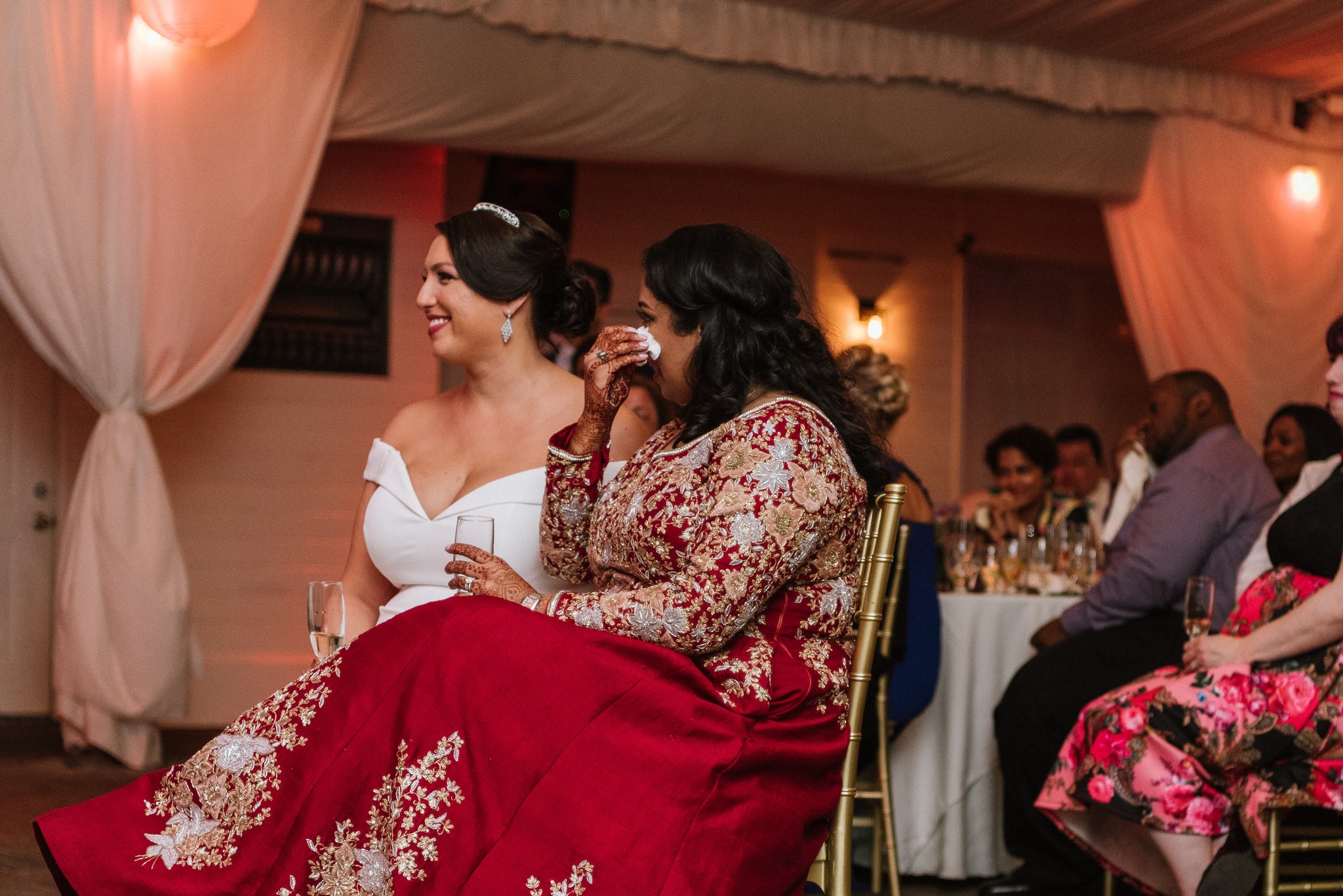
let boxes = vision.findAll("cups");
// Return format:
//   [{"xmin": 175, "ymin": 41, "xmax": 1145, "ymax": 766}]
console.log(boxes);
[
  {"xmin": 941, "ymin": 521, "xmax": 1097, "ymax": 596},
  {"xmin": 306, "ymin": 580, "xmax": 346, "ymax": 662},
  {"xmin": 452, "ymin": 514, "xmax": 494, "ymax": 595},
  {"xmin": 1183, "ymin": 576, "xmax": 1215, "ymax": 638}
]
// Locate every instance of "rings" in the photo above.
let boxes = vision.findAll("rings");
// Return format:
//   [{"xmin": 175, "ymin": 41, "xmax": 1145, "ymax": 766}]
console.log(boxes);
[
  {"xmin": 596, "ymin": 349, "xmax": 609, "ymax": 361},
  {"xmin": 464, "ymin": 576, "xmax": 475, "ymax": 593},
  {"xmin": 1190, "ymin": 659, "xmax": 1197, "ymax": 671}
]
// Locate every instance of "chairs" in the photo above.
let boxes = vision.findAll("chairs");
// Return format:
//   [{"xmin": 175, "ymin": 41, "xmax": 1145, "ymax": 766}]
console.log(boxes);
[
  {"xmin": 852, "ymin": 524, "xmax": 910, "ymax": 896},
  {"xmin": 821, "ymin": 482, "xmax": 908, "ymax": 896},
  {"xmin": 1265, "ymin": 806, "xmax": 1343, "ymax": 896}
]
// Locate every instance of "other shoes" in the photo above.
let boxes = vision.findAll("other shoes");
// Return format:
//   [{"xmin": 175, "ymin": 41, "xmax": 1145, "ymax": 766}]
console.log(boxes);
[{"xmin": 992, "ymin": 860, "xmax": 1105, "ymax": 896}]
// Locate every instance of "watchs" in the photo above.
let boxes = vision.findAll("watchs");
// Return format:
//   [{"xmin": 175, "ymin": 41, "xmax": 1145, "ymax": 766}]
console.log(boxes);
[{"xmin": 521, "ymin": 592, "xmax": 544, "ymax": 611}]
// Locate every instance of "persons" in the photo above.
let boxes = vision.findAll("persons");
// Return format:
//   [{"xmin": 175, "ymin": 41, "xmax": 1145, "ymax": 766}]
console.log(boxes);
[
  {"xmin": 544, "ymin": 260, "xmax": 615, "ymax": 388},
  {"xmin": 976, "ymin": 369, "xmax": 1279, "ymax": 896},
  {"xmin": 1050, "ymin": 422, "xmax": 1116, "ymax": 520},
  {"xmin": 986, "ymin": 422, "xmax": 1088, "ymax": 539},
  {"xmin": 1262, "ymin": 401, "xmax": 1343, "ymax": 498},
  {"xmin": 822, "ymin": 345, "xmax": 942, "ymax": 768},
  {"xmin": 29, "ymin": 219, "xmax": 868, "ymax": 895},
  {"xmin": 1031, "ymin": 315, "xmax": 1343, "ymax": 896},
  {"xmin": 308, "ymin": 205, "xmax": 659, "ymax": 667}
]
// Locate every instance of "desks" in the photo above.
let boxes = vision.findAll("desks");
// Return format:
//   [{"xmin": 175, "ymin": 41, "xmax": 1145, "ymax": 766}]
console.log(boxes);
[{"xmin": 887, "ymin": 593, "xmax": 1085, "ymax": 880}]
